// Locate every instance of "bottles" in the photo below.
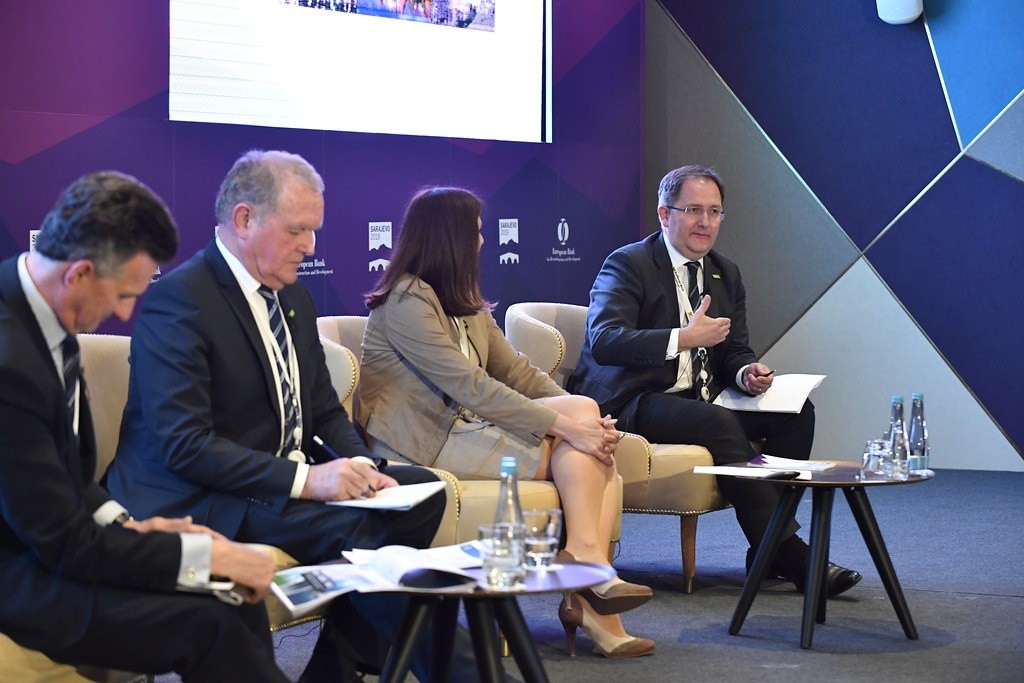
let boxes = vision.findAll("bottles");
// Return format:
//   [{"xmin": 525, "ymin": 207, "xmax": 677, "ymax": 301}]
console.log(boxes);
[
  {"xmin": 495, "ymin": 456, "xmax": 523, "ymax": 524},
  {"xmin": 908, "ymin": 392, "xmax": 930, "ymax": 476},
  {"xmin": 882, "ymin": 395, "xmax": 910, "ymax": 460}
]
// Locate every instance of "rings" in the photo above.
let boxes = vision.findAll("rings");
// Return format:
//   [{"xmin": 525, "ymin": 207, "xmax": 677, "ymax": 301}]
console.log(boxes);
[{"xmin": 759, "ymin": 389, "xmax": 761, "ymax": 395}]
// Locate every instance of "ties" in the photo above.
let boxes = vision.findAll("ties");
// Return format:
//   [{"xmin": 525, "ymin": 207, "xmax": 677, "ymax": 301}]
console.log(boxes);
[
  {"xmin": 60, "ymin": 331, "xmax": 80, "ymax": 425},
  {"xmin": 257, "ymin": 284, "xmax": 301, "ymax": 458},
  {"xmin": 684, "ymin": 260, "xmax": 716, "ymax": 403}
]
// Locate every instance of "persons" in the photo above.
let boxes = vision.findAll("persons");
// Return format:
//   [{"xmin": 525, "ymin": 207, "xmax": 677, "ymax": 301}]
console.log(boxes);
[
  {"xmin": 351, "ymin": 186, "xmax": 657, "ymax": 660},
  {"xmin": 566, "ymin": 164, "xmax": 862, "ymax": 599},
  {"xmin": 0, "ymin": 172, "xmax": 293, "ymax": 683},
  {"xmin": 98, "ymin": 148, "xmax": 523, "ymax": 683}
]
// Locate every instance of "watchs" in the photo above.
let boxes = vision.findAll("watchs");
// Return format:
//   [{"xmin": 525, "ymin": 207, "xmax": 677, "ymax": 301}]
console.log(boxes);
[{"xmin": 111, "ymin": 512, "xmax": 134, "ymax": 529}]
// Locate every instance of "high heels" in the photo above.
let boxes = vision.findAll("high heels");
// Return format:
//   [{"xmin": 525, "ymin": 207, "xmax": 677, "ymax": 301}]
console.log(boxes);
[
  {"xmin": 553, "ymin": 550, "xmax": 653, "ymax": 616},
  {"xmin": 558, "ymin": 592, "xmax": 655, "ymax": 659}
]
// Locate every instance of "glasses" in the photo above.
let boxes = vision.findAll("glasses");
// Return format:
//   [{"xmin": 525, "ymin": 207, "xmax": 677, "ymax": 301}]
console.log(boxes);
[{"xmin": 666, "ymin": 205, "xmax": 725, "ymax": 222}]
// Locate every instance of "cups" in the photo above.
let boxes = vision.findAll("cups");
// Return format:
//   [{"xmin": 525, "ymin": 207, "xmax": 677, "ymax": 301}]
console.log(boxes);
[
  {"xmin": 523, "ymin": 508, "xmax": 563, "ymax": 571},
  {"xmin": 859, "ymin": 440, "xmax": 912, "ymax": 481},
  {"xmin": 479, "ymin": 523, "xmax": 527, "ymax": 588}
]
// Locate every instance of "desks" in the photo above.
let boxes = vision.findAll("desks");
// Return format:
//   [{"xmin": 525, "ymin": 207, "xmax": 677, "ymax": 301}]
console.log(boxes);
[
  {"xmin": 354, "ymin": 561, "xmax": 609, "ymax": 683},
  {"xmin": 729, "ymin": 462, "xmax": 934, "ymax": 648}
]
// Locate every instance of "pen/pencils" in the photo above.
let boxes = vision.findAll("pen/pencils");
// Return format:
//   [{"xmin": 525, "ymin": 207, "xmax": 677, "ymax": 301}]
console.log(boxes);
[
  {"xmin": 764, "ymin": 369, "xmax": 776, "ymax": 377},
  {"xmin": 313, "ymin": 435, "xmax": 339, "ymax": 459}
]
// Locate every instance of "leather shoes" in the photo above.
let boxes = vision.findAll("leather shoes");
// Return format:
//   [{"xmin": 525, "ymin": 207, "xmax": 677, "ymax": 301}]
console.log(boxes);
[
  {"xmin": 790, "ymin": 544, "xmax": 863, "ymax": 597},
  {"xmin": 745, "ymin": 548, "xmax": 792, "ymax": 580}
]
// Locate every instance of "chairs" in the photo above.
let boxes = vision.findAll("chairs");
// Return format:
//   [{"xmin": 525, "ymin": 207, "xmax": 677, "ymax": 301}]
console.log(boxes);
[
  {"xmin": 504, "ymin": 300, "xmax": 739, "ymax": 595},
  {"xmin": 314, "ymin": 315, "xmax": 620, "ymax": 659},
  {"xmin": 71, "ymin": 335, "xmax": 143, "ymax": 491}
]
